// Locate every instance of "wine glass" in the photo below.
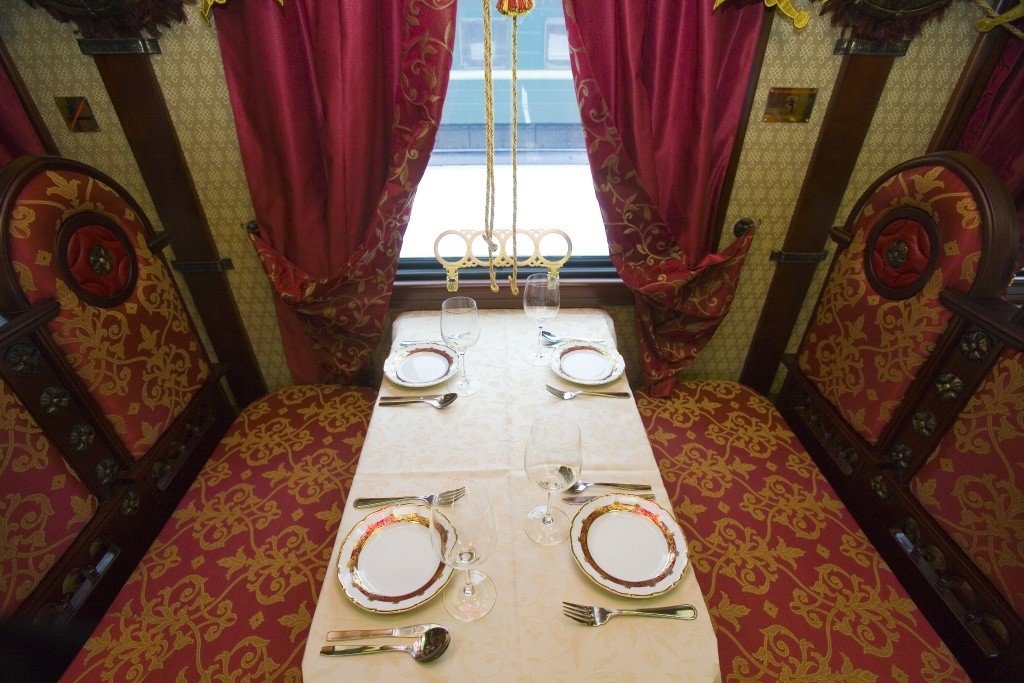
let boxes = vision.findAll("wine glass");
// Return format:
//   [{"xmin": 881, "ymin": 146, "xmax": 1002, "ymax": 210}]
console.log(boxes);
[
  {"xmin": 523, "ymin": 414, "xmax": 582, "ymax": 547},
  {"xmin": 522, "ymin": 273, "xmax": 560, "ymax": 366},
  {"xmin": 440, "ymin": 296, "xmax": 482, "ymax": 397},
  {"xmin": 429, "ymin": 478, "xmax": 498, "ymax": 622}
]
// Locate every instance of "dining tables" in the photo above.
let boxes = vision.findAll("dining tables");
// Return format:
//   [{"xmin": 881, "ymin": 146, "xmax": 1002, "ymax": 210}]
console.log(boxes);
[{"xmin": 302, "ymin": 308, "xmax": 721, "ymax": 683}]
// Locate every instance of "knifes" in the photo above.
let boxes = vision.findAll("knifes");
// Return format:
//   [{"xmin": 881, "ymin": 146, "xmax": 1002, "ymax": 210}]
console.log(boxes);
[
  {"xmin": 561, "ymin": 493, "xmax": 655, "ymax": 505},
  {"xmin": 381, "ymin": 395, "xmax": 443, "ymax": 400},
  {"xmin": 325, "ymin": 622, "xmax": 451, "ymax": 642}
]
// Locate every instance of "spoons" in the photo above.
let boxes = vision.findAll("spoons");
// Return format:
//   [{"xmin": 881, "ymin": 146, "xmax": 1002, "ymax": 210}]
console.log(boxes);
[
  {"xmin": 378, "ymin": 392, "xmax": 457, "ymax": 409},
  {"xmin": 561, "ymin": 482, "xmax": 651, "ymax": 494},
  {"xmin": 539, "ymin": 330, "xmax": 606, "ymax": 343},
  {"xmin": 320, "ymin": 627, "xmax": 451, "ymax": 663}
]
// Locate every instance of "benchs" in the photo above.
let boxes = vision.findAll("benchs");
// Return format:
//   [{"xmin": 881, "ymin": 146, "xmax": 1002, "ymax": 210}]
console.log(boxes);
[
  {"xmin": 0, "ymin": 160, "xmax": 382, "ymax": 683},
  {"xmin": 632, "ymin": 157, "xmax": 1024, "ymax": 683}
]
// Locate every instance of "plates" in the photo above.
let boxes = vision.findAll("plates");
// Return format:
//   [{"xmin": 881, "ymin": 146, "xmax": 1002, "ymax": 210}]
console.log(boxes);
[
  {"xmin": 549, "ymin": 342, "xmax": 626, "ymax": 385},
  {"xmin": 383, "ymin": 343, "xmax": 460, "ymax": 388},
  {"xmin": 336, "ymin": 504, "xmax": 457, "ymax": 614},
  {"xmin": 568, "ymin": 493, "xmax": 690, "ymax": 599}
]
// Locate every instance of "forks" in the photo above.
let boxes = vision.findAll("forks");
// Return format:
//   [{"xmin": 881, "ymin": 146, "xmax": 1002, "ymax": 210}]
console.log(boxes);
[
  {"xmin": 353, "ymin": 487, "xmax": 466, "ymax": 509},
  {"xmin": 545, "ymin": 384, "xmax": 630, "ymax": 400},
  {"xmin": 561, "ymin": 601, "xmax": 698, "ymax": 627}
]
